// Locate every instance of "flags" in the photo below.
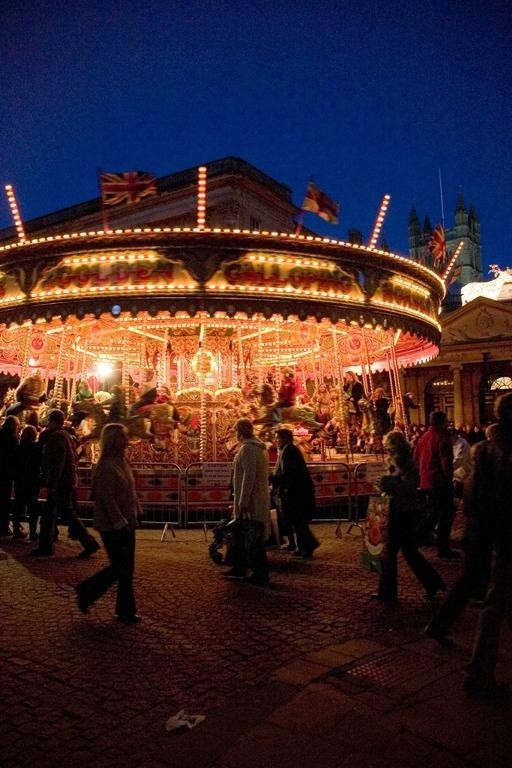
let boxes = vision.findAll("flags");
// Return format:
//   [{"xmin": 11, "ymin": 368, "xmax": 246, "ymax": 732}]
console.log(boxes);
[
  {"xmin": 424, "ymin": 222, "xmax": 448, "ymax": 265},
  {"xmin": 98, "ymin": 168, "xmax": 165, "ymax": 206},
  {"xmin": 302, "ymin": 183, "xmax": 342, "ymax": 226}
]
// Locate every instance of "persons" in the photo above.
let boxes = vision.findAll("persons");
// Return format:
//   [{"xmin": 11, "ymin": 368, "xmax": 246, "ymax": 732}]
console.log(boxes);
[
  {"xmin": 220, "ymin": 418, "xmax": 270, "ymax": 584},
  {"xmin": 266, "ymin": 429, "xmax": 321, "ymax": 555},
  {"xmin": 1, "ymin": 406, "xmax": 101, "ymax": 560},
  {"xmin": 72, "ymin": 423, "xmax": 143, "ymax": 623},
  {"xmin": 1, "ymin": 322, "xmax": 420, "ymax": 466}
]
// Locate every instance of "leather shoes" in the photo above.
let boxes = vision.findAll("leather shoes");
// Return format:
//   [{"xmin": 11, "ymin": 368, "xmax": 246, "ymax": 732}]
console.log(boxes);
[
  {"xmin": 415, "ymin": 605, "xmax": 424, "ymax": 615},
  {"xmin": 78, "ymin": 548, "xmax": 92, "ymax": 559},
  {"xmin": 425, "ymin": 625, "xmax": 453, "ymax": 648},
  {"xmin": 117, "ymin": 615, "xmax": 142, "ymax": 623},
  {"xmin": 29, "ymin": 546, "xmax": 52, "ymax": 557}
]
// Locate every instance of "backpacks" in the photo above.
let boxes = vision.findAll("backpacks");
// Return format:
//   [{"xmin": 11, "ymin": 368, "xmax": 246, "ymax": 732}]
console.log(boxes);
[{"xmin": 409, "ymin": 489, "xmax": 439, "ymax": 544}]
